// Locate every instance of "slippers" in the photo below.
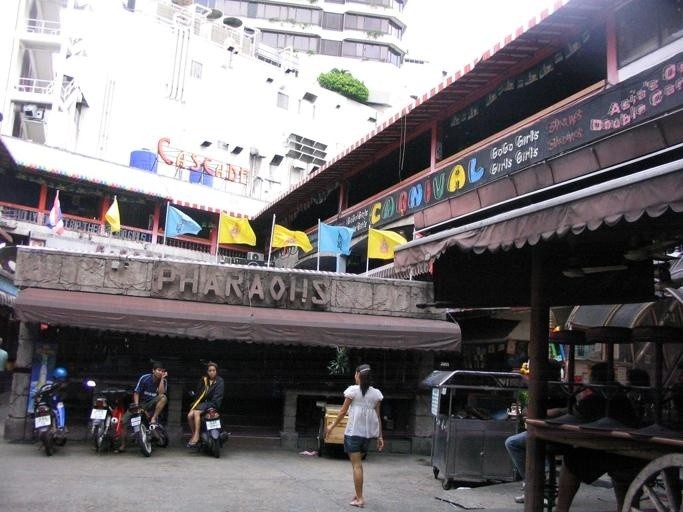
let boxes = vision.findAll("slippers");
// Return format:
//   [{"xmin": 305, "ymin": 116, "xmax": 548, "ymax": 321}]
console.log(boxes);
[
  {"xmin": 187, "ymin": 438, "xmax": 200, "ymax": 448},
  {"xmin": 151, "ymin": 420, "xmax": 156, "ymax": 424},
  {"xmin": 114, "ymin": 448, "xmax": 126, "ymax": 454}
]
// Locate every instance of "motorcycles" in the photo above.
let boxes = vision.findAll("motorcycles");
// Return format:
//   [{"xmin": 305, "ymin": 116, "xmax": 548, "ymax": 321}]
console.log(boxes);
[{"xmin": 26, "ymin": 370, "xmax": 231, "ymax": 459}]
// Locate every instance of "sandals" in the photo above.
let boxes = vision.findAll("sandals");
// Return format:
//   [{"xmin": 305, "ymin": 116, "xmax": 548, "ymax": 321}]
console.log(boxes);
[{"xmin": 350, "ymin": 496, "xmax": 365, "ymax": 508}]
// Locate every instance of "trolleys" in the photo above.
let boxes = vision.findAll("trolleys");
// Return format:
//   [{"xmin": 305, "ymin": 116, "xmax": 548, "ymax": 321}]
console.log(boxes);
[{"xmin": 312, "ymin": 401, "xmax": 369, "ymax": 462}]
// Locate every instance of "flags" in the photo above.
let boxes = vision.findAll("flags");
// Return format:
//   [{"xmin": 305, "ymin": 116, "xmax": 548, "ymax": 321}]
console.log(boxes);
[
  {"xmin": 217, "ymin": 209, "xmax": 257, "ymax": 246},
  {"xmin": 45, "ymin": 189, "xmax": 64, "ymax": 235},
  {"xmin": 271, "ymin": 222, "xmax": 409, "ymax": 260},
  {"xmin": 105, "ymin": 195, "xmax": 121, "ymax": 234},
  {"xmin": 165, "ymin": 199, "xmax": 203, "ymax": 238}
]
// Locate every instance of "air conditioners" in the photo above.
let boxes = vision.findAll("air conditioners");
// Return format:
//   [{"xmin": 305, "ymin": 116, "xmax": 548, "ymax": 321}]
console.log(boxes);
[{"xmin": 247, "ymin": 252, "xmax": 264, "ymax": 260}]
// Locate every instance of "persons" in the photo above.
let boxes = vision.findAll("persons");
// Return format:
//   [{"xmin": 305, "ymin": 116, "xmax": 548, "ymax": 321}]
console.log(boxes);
[
  {"xmin": 504, "ymin": 361, "xmax": 655, "ymax": 512},
  {"xmin": 325, "ymin": 365, "xmax": 384, "ymax": 508},
  {"xmin": 185, "ymin": 361, "xmax": 225, "ymax": 449},
  {"xmin": 0, "ymin": 337, "xmax": 8, "ymax": 406},
  {"xmin": 113, "ymin": 361, "xmax": 169, "ymax": 454}
]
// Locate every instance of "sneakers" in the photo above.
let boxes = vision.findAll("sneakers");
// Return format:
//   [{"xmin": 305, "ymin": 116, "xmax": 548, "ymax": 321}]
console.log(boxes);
[{"xmin": 515, "ymin": 496, "xmax": 525, "ymax": 503}]
